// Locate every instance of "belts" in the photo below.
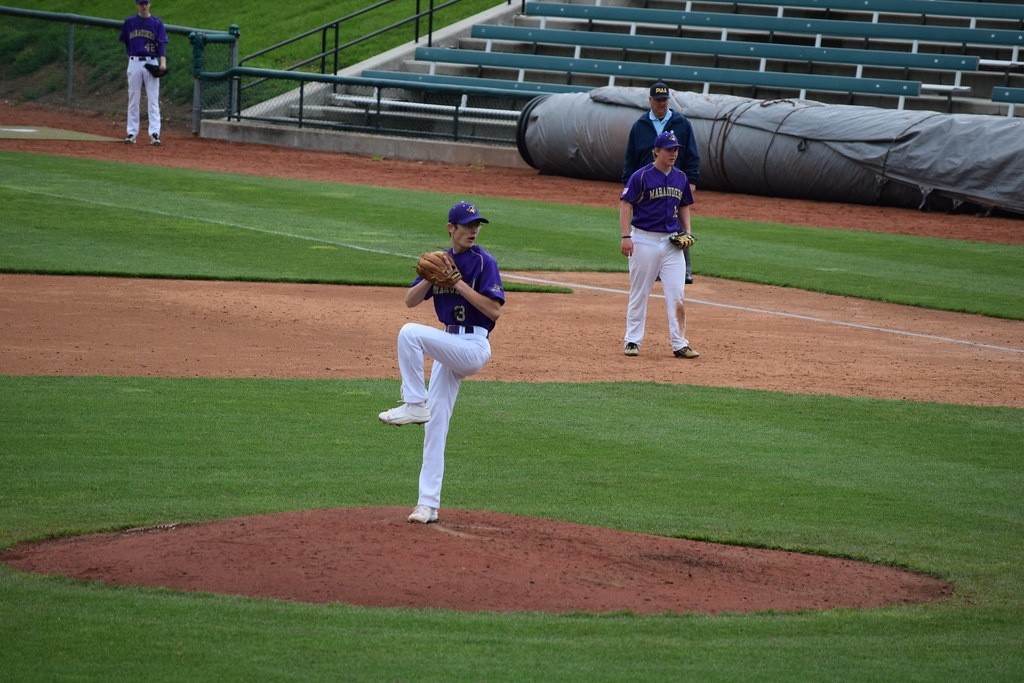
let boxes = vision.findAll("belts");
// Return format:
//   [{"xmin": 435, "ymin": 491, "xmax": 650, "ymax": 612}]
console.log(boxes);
[
  {"xmin": 445, "ymin": 324, "xmax": 489, "ymax": 340},
  {"xmin": 131, "ymin": 56, "xmax": 154, "ymax": 61}
]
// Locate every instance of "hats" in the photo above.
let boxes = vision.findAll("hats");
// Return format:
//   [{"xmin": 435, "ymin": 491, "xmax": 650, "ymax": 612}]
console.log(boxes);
[
  {"xmin": 654, "ymin": 132, "xmax": 683, "ymax": 151},
  {"xmin": 449, "ymin": 201, "xmax": 489, "ymax": 225},
  {"xmin": 136, "ymin": 0, "xmax": 150, "ymax": 5},
  {"xmin": 650, "ymin": 83, "xmax": 670, "ymax": 100}
]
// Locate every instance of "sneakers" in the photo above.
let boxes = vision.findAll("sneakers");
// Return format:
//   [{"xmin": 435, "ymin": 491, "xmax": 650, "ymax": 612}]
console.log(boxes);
[
  {"xmin": 673, "ymin": 346, "xmax": 699, "ymax": 358},
  {"xmin": 407, "ymin": 505, "xmax": 438, "ymax": 524},
  {"xmin": 125, "ymin": 134, "xmax": 136, "ymax": 144},
  {"xmin": 378, "ymin": 400, "xmax": 430, "ymax": 427},
  {"xmin": 150, "ymin": 133, "xmax": 161, "ymax": 145},
  {"xmin": 624, "ymin": 342, "xmax": 639, "ymax": 356}
]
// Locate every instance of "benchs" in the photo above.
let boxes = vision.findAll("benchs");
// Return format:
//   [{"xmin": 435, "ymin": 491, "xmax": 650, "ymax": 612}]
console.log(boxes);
[{"xmin": 288, "ymin": 0, "xmax": 1024, "ymax": 147}]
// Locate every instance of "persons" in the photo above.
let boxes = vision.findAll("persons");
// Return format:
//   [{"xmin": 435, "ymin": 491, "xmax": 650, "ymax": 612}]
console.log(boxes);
[
  {"xmin": 619, "ymin": 132, "xmax": 700, "ymax": 359},
  {"xmin": 118, "ymin": 0, "xmax": 169, "ymax": 146},
  {"xmin": 622, "ymin": 82, "xmax": 701, "ymax": 284},
  {"xmin": 378, "ymin": 202, "xmax": 506, "ymax": 524}
]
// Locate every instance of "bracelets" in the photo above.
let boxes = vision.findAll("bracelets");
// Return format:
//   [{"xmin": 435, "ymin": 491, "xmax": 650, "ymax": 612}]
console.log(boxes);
[{"xmin": 621, "ymin": 236, "xmax": 631, "ymax": 238}]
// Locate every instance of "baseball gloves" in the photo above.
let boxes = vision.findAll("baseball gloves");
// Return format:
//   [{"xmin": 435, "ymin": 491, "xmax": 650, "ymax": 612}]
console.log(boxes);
[
  {"xmin": 668, "ymin": 232, "xmax": 698, "ymax": 250},
  {"xmin": 415, "ymin": 250, "xmax": 462, "ymax": 291}
]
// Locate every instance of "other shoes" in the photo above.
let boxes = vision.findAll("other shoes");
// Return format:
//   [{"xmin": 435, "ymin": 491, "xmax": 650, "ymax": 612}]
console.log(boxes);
[{"xmin": 685, "ymin": 274, "xmax": 693, "ymax": 284}]
